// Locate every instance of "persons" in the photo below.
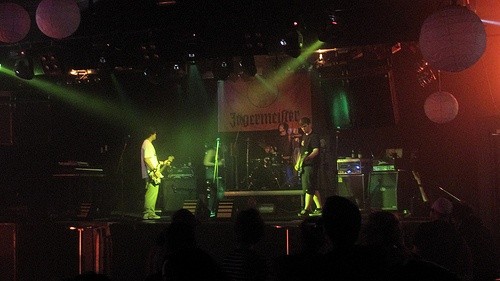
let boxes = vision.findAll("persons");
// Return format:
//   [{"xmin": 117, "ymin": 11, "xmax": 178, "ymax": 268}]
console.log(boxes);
[
  {"xmin": 272, "ymin": 217, "xmax": 330, "ymax": 281},
  {"xmin": 294, "ymin": 117, "xmax": 324, "ymax": 216},
  {"xmin": 156, "ymin": 209, "xmax": 226, "ymax": 281},
  {"xmin": 265, "ymin": 122, "xmax": 295, "ymax": 187},
  {"xmin": 141, "ymin": 127, "xmax": 170, "ymax": 220},
  {"xmin": 367, "ymin": 211, "xmax": 417, "ymax": 260},
  {"xmin": 203, "ymin": 142, "xmax": 225, "ymax": 217},
  {"xmin": 321, "ymin": 197, "xmax": 374, "ymax": 281},
  {"xmin": 220, "ymin": 208, "xmax": 281, "ymax": 281}
]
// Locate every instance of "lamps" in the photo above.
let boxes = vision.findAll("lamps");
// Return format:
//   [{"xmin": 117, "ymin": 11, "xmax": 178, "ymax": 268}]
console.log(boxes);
[{"xmin": 15, "ymin": 8, "xmax": 347, "ymax": 83}]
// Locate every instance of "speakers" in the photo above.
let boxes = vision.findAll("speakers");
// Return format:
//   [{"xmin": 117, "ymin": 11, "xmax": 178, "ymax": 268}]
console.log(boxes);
[
  {"xmin": 367, "ymin": 172, "xmax": 398, "ymax": 210},
  {"xmin": 163, "ymin": 174, "xmax": 197, "ymax": 212},
  {"xmin": 337, "ymin": 174, "xmax": 365, "ymax": 211}
]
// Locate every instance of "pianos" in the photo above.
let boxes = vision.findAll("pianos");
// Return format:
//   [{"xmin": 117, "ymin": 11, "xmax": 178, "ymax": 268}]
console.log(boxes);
[{"xmin": 48, "ymin": 159, "xmax": 114, "ymax": 220}]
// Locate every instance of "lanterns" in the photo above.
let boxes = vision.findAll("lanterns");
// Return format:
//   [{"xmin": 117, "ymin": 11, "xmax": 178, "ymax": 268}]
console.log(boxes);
[
  {"xmin": 36, "ymin": 0, "xmax": 80, "ymax": 38},
  {"xmin": 419, "ymin": 4, "xmax": 485, "ymax": 72},
  {"xmin": 0, "ymin": 3, "xmax": 31, "ymax": 44},
  {"xmin": 425, "ymin": 93, "xmax": 458, "ymax": 124}
]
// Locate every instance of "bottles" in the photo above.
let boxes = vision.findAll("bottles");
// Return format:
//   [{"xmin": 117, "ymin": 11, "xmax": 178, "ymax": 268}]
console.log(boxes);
[
  {"xmin": 352, "ymin": 148, "xmax": 355, "ymax": 159},
  {"xmin": 358, "ymin": 148, "xmax": 362, "ymax": 159}
]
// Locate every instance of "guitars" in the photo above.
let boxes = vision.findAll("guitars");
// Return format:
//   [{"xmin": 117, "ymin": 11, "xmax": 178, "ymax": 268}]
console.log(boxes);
[
  {"xmin": 148, "ymin": 154, "xmax": 176, "ymax": 185},
  {"xmin": 294, "ymin": 149, "xmax": 310, "ymax": 174}
]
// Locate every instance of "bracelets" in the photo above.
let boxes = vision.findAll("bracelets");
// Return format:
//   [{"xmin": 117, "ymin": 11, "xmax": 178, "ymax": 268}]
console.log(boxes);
[{"xmin": 153, "ymin": 169, "xmax": 157, "ymax": 173}]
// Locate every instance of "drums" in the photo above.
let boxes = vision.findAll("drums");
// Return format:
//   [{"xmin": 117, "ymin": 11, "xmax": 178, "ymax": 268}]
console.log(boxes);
[
  {"xmin": 269, "ymin": 154, "xmax": 284, "ymax": 168},
  {"xmin": 250, "ymin": 156, "xmax": 265, "ymax": 171}
]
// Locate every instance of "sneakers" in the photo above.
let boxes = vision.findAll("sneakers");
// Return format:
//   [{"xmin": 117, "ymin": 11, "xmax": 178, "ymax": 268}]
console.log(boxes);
[
  {"xmin": 298, "ymin": 210, "xmax": 310, "ymax": 216},
  {"xmin": 143, "ymin": 212, "xmax": 160, "ymax": 219},
  {"xmin": 309, "ymin": 208, "xmax": 323, "ymax": 216}
]
